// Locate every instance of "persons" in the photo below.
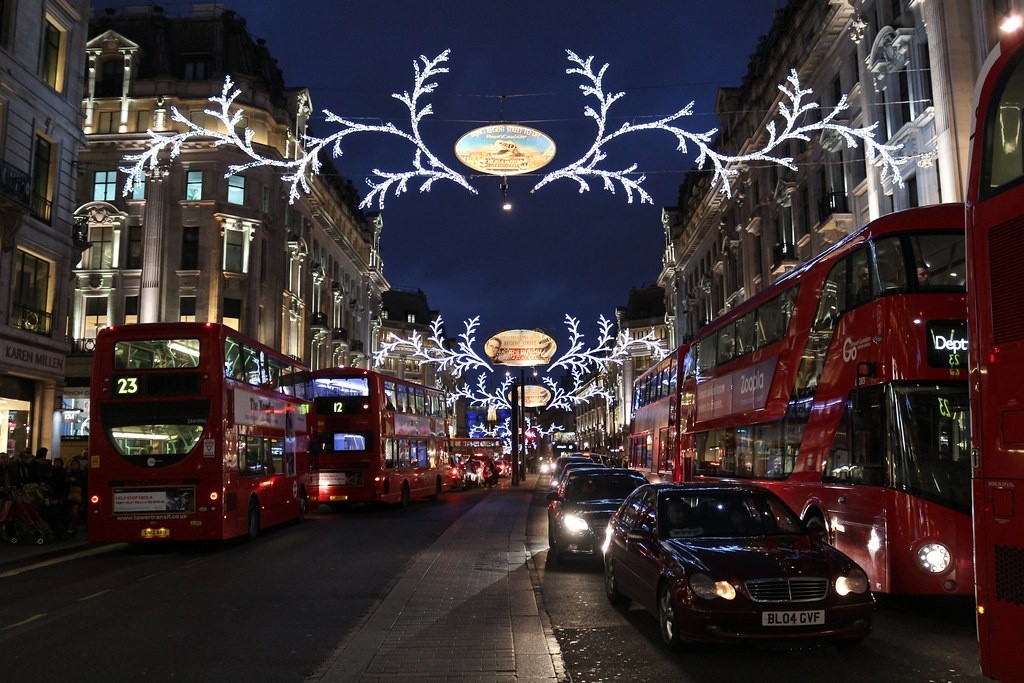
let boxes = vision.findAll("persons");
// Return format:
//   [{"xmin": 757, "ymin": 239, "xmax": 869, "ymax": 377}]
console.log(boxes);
[
  {"xmin": 572, "ymin": 479, "xmax": 602, "ymax": 501},
  {"xmin": 913, "ymin": 261, "xmax": 932, "ymax": 288},
  {"xmin": 0, "ymin": 448, "xmax": 88, "ymax": 535},
  {"xmin": 714, "ymin": 502, "xmax": 756, "ymax": 537},
  {"xmin": 659, "ymin": 497, "xmax": 707, "ymax": 538},
  {"xmin": 854, "ymin": 265, "xmax": 897, "ymax": 305}
]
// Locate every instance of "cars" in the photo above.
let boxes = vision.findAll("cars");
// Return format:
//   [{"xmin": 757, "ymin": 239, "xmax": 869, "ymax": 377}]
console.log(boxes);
[
  {"xmin": 550, "ymin": 453, "xmax": 601, "ymax": 486},
  {"xmin": 545, "ymin": 467, "xmax": 650, "ymax": 563},
  {"xmin": 559, "ymin": 463, "xmax": 606, "ymax": 482},
  {"xmin": 603, "ymin": 482, "xmax": 875, "ymax": 649},
  {"xmin": 494, "ymin": 460, "xmax": 510, "ymax": 477},
  {"xmin": 461, "ymin": 459, "xmax": 485, "ymax": 488}
]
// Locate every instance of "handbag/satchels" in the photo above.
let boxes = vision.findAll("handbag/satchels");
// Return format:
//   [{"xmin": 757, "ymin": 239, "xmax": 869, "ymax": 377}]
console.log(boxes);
[{"xmin": 68, "ymin": 487, "xmax": 82, "ymax": 502}]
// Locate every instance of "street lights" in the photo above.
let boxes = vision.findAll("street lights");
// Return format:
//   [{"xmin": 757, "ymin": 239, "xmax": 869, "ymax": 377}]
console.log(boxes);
[
  {"xmin": 487, "ymin": 404, "xmax": 496, "ymax": 432},
  {"xmin": 484, "ymin": 329, "xmax": 557, "ymax": 481},
  {"xmin": 507, "ymin": 385, "xmax": 551, "ymax": 481}
]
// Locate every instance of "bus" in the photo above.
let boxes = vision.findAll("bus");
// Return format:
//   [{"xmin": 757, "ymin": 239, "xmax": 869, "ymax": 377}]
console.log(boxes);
[
  {"xmin": 450, "ymin": 438, "xmax": 503, "ymax": 461},
  {"xmin": 680, "ymin": 202, "xmax": 975, "ymax": 595},
  {"xmin": 966, "ymin": 31, "xmax": 1024, "ymax": 683},
  {"xmin": 311, "ymin": 368, "xmax": 452, "ymax": 503},
  {"xmin": 627, "ymin": 344, "xmax": 690, "ymax": 483},
  {"xmin": 87, "ymin": 322, "xmax": 316, "ymax": 542}
]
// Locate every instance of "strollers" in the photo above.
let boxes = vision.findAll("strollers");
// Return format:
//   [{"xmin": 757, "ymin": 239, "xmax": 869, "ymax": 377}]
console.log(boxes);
[{"xmin": 3, "ymin": 486, "xmax": 53, "ymax": 545}]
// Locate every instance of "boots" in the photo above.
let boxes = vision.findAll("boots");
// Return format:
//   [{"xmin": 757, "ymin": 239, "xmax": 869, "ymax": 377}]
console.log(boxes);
[{"xmin": 1, "ymin": 529, "xmax": 9, "ymax": 540}]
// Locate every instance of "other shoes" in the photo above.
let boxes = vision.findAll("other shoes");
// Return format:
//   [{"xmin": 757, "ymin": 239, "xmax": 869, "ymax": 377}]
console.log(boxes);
[{"xmin": 68, "ymin": 528, "xmax": 78, "ymax": 533}]
